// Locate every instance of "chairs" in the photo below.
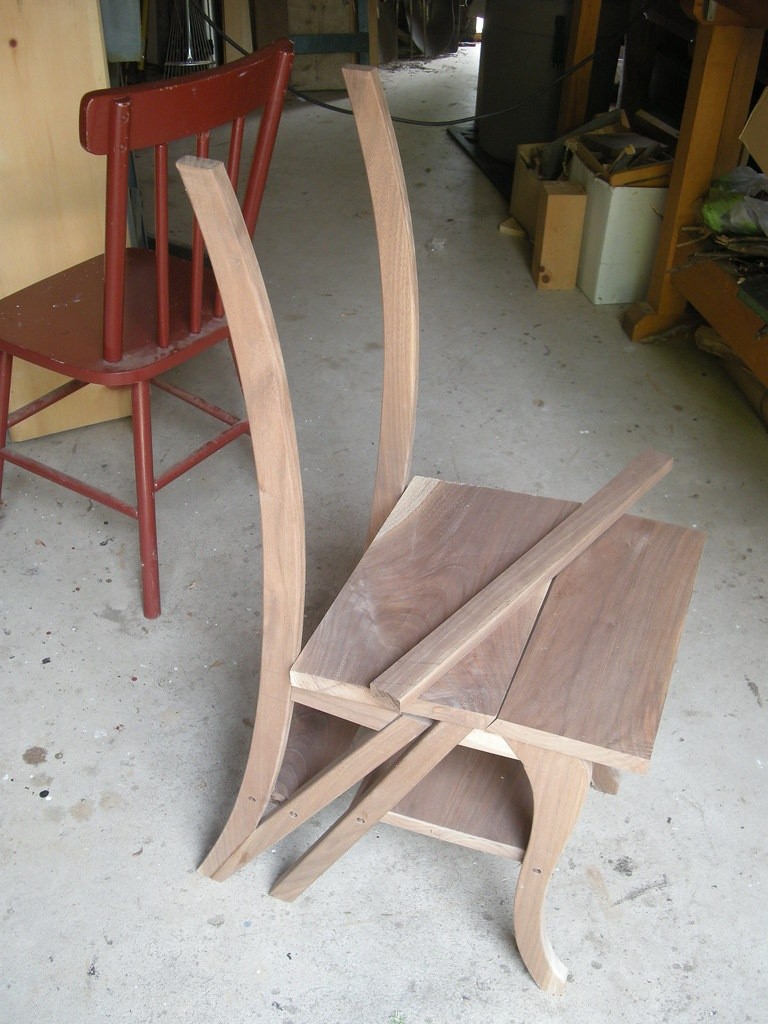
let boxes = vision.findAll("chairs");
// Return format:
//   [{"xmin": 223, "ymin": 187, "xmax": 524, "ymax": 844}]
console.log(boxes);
[
  {"xmin": 175, "ymin": 63, "xmax": 705, "ymax": 992},
  {"xmin": 0, "ymin": 34, "xmax": 297, "ymax": 619}
]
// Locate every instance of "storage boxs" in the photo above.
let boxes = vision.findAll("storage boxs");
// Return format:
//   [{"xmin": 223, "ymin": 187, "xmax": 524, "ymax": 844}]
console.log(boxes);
[
  {"xmin": 559, "ymin": 132, "xmax": 674, "ymax": 305},
  {"xmin": 508, "ymin": 142, "xmax": 568, "ymax": 242}
]
